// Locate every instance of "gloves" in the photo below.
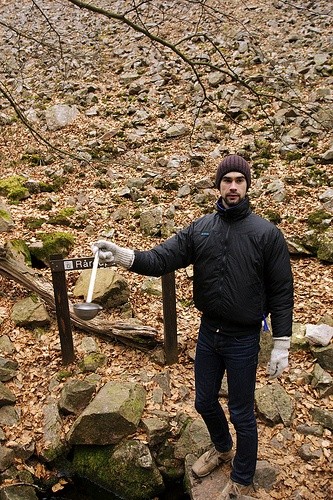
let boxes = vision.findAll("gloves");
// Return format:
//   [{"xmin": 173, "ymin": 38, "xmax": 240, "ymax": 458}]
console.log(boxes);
[
  {"xmin": 91, "ymin": 240, "xmax": 134, "ymax": 269},
  {"xmin": 268, "ymin": 336, "xmax": 291, "ymax": 381}
]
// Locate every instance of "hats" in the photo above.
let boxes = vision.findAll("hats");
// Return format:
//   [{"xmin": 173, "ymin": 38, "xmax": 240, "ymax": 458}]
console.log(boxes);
[{"xmin": 216, "ymin": 155, "xmax": 251, "ymax": 193}]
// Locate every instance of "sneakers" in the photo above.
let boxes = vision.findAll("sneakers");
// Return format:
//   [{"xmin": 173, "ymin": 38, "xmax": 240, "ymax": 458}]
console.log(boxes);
[
  {"xmin": 191, "ymin": 445, "xmax": 236, "ymax": 477},
  {"xmin": 216, "ymin": 480, "xmax": 252, "ymax": 500}
]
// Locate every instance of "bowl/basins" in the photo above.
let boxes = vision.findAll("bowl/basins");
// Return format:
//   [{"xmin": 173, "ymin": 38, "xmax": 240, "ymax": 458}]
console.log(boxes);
[{"xmin": 74, "ymin": 302, "xmax": 103, "ymax": 320}]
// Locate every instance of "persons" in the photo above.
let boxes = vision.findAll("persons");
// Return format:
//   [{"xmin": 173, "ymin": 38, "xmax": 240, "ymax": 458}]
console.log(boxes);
[{"xmin": 91, "ymin": 155, "xmax": 294, "ymax": 500}]
